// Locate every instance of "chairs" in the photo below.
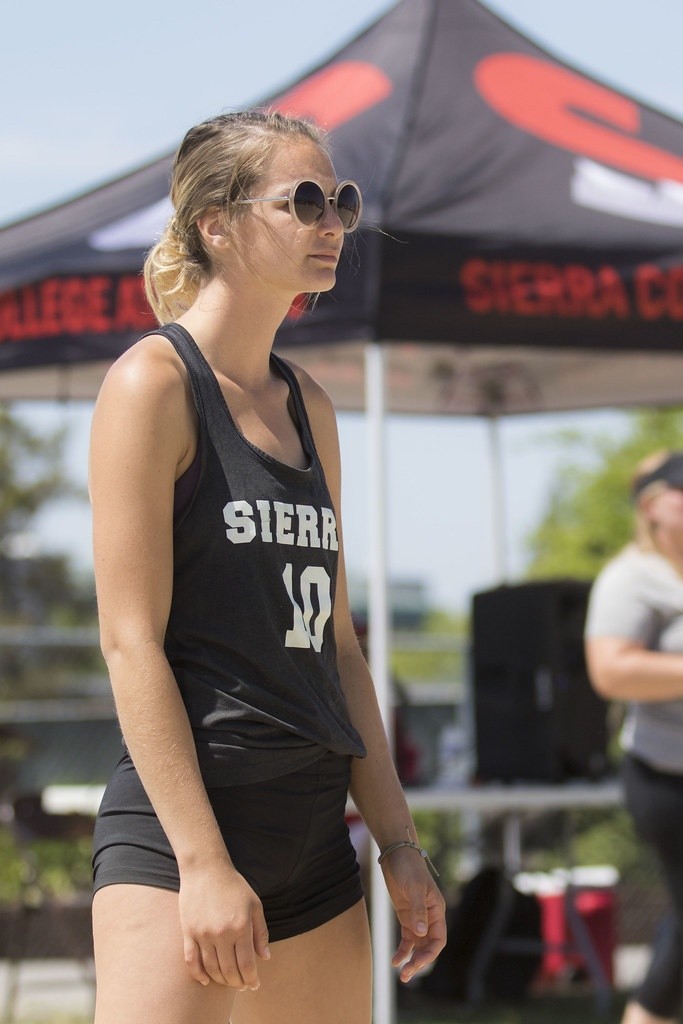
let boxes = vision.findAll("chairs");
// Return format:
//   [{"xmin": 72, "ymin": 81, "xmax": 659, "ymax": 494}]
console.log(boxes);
[{"xmin": 3, "ymin": 787, "xmax": 98, "ymax": 1024}]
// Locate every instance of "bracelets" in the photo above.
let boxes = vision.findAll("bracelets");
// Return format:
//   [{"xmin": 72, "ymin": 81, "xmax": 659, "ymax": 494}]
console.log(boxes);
[{"xmin": 374, "ymin": 830, "xmax": 440, "ymax": 879}]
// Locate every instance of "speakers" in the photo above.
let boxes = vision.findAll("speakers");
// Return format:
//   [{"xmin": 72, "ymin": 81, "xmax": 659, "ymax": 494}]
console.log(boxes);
[{"xmin": 473, "ymin": 577, "xmax": 611, "ymax": 788}]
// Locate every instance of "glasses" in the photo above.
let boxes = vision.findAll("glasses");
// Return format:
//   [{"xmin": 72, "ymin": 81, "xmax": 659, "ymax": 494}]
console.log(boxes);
[{"xmin": 231, "ymin": 177, "xmax": 362, "ymax": 233}]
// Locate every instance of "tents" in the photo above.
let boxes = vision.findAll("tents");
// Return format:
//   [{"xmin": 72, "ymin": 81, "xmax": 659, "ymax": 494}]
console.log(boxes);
[{"xmin": 0, "ymin": 0, "xmax": 683, "ymax": 1024}]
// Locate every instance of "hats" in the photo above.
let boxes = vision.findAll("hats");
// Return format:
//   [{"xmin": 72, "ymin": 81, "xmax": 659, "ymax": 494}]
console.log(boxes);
[{"xmin": 635, "ymin": 452, "xmax": 683, "ymax": 491}]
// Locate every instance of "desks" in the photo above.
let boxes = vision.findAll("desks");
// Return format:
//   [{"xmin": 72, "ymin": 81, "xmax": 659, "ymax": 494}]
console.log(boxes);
[{"xmin": 38, "ymin": 781, "xmax": 626, "ymax": 1024}]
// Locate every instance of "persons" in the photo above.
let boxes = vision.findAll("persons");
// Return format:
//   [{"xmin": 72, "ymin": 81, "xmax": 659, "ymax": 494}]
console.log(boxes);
[
  {"xmin": 583, "ymin": 450, "xmax": 683, "ymax": 1024},
  {"xmin": 86, "ymin": 115, "xmax": 446, "ymax": 1024}
]
